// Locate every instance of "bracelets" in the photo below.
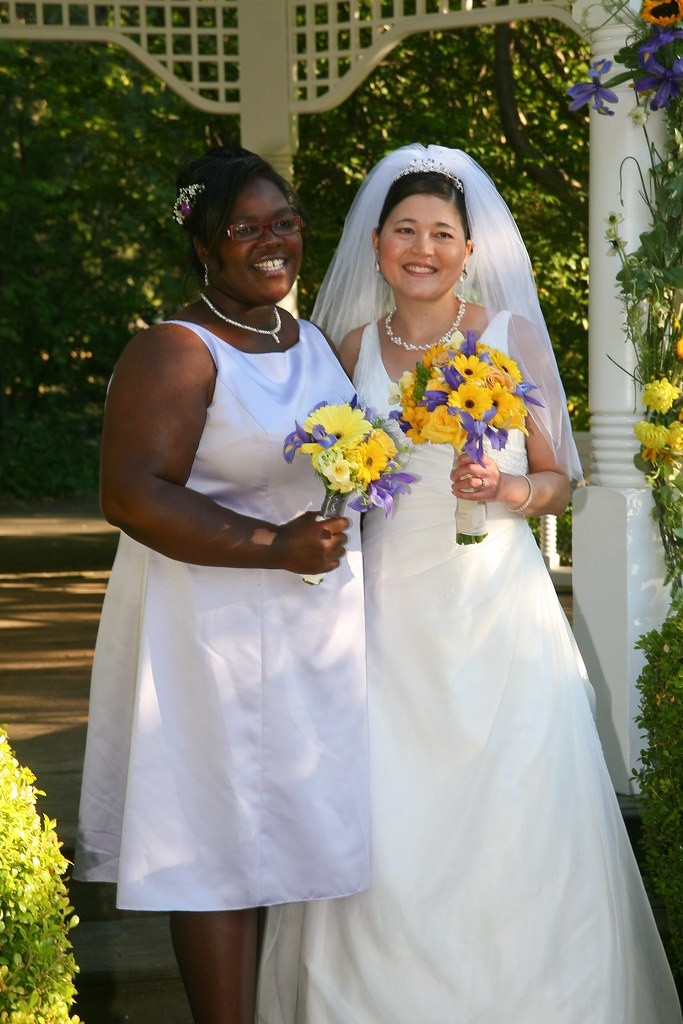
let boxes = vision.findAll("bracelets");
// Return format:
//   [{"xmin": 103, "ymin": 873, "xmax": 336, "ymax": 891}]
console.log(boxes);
[{"xmin": 507, "ymin": 475, "xmax": 533, "ymax": 513}]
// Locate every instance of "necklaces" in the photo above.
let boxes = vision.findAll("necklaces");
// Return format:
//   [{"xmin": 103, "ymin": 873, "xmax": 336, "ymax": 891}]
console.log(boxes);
[
  {"xmin": 198, "ymin": 291, "xmax": 282, "ymax": 345},
  {"xmin": 384, "ymin": 294, "xmax": 466, "ymax": 351}
]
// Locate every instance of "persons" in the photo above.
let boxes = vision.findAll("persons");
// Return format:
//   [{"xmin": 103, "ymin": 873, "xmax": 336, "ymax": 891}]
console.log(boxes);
[
  {"xmin": 71, "ymin": 144, "xmax": 366, "ymax": 1024},
  {"xmin": 255, "ymin": 142, "xmax": 683, "ymax": 1024}
]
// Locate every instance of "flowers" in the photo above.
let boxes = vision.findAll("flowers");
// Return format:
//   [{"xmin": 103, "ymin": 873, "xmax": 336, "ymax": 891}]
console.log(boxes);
[
  {"xmin": 282, "ymin": 394, "xmax": 420, "ymax": 581},
  {"xmin": 384, "ymin": 333, "xmax": 545, "ymax": 543},
  {"xmin": 170, "ymin": 184, "xmax": 206, "ymax": 226}
]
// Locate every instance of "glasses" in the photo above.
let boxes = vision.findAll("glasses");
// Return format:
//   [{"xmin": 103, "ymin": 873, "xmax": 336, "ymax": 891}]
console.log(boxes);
[{"xmin": 218, "ymin": 215, "xmax": 304, "ymax": 242}]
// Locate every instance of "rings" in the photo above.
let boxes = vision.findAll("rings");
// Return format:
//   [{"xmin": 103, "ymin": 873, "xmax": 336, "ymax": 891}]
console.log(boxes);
[{"xmin": 480, "ymin": 478, "xmax": 488, "ymax": 489}]
